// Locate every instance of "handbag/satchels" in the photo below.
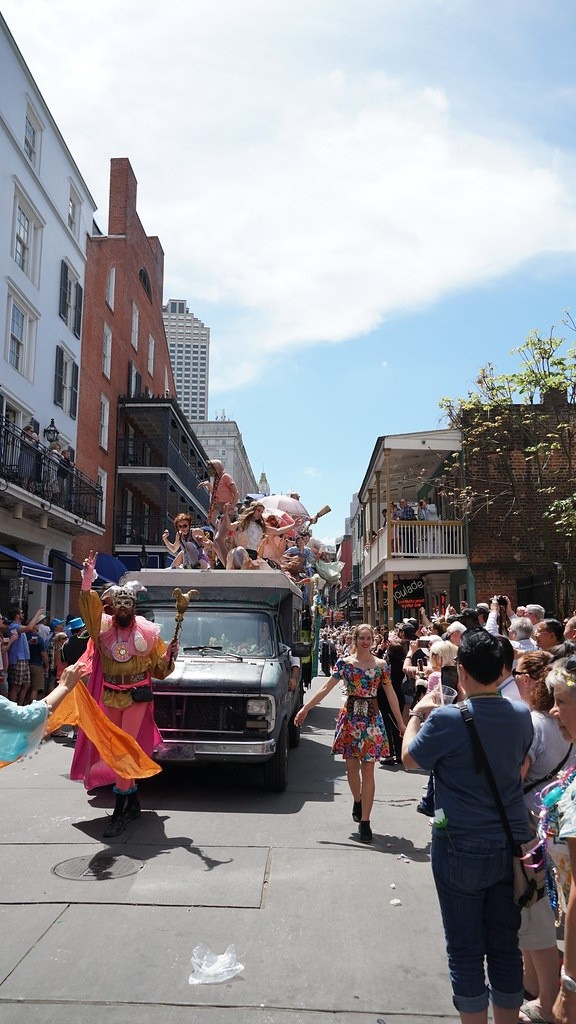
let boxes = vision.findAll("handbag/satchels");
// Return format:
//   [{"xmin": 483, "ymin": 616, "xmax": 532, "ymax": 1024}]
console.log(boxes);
[{"xmin": 514, "ymin": 839, "xmax": 548, "ymax": 907}]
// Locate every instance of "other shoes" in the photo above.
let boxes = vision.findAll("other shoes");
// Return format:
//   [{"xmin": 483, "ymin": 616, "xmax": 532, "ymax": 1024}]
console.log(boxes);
[
  {"xmin": 352, "ymin": 799, "xmax": 363, "ymax": 822},
  {"xmin": 51, "ymin": 729, "xmax": 70, "ymax": 738},
  {"xmin": 358, "ymin": 820, "xmax": 373, "ymax": 843}
]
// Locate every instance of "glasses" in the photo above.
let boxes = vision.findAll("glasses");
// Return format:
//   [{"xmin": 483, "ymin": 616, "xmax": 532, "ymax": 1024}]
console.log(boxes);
[
  {"xmin": 176, "ymin": 523, "xmax": 189, "ymax": 529},
  {"xmin": 62, "ymin": 637, "xmax": 68, "ymax": 640},
  {"xmin": 0, "ymin": 617, "xmax": 4, "ymax": 620},
  {"xmin": 447, "ymin": 632, "xmax": 456, "ymax": 641},
  {"xmin": 19, "ymin": 611, "xmax": 24, "ymax": 615},
  {"xmin": 511, "ymin": 667, "xmax": 537, "ymax": 681},
  {"xmin": 565, "ymin": 655, "xmax": 576, "ymax": 673}
]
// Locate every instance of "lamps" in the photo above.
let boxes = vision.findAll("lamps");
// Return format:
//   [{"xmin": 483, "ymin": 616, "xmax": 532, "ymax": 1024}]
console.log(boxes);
[
  {"xmin": 43, "ymin": 418, "xmax": 59, "ymax": 444},
  {"xmin": 39, "ymin": 515, "xmax": 48, "ymax": 529},
  {"xmin": 13, "ymin": 502, "xmax": 23, "ymax": 519}
]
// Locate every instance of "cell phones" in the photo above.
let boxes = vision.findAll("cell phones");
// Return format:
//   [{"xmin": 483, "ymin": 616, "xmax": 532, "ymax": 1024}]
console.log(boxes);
[
  {"xmin": 418, "ymin": 640, "xmax": 429, "ymax": 648},
  {"xmin": 441, "ymin": 665, "xmax": 458, "ymax": 704},
  {"xmin": 384, "ymin": 631, "xmax": 389, "ymax": 642}
]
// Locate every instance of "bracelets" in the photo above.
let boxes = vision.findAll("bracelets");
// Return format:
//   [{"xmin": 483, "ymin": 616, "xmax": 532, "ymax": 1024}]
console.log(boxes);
[{"xmin": 404, "ymin": 656, "xmax": 412, "ymax": 659}]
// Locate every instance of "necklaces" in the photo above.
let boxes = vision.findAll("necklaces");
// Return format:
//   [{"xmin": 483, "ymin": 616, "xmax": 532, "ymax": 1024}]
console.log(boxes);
[
  {"xmin": 206, "ymin": 473, "xmax": 224, "ymax": 524},
  {"xmin": 112, "ymin": 619, "xmax": 135, "ymax": 663},
  {"xmin": 541, "ymin": 769, "xmax": 576, "ymax": 909},
  {"xmin": 355, "ymin": 650, "xmax": 373, "ymax": 670}
]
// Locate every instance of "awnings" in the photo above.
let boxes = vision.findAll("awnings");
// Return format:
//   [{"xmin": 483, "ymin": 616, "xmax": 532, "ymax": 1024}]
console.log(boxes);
[
  {"xmin": 0, "ymin": 546, "xmax": 54, "ymax": 582},
  {"xmin": 51, "ymin": 549, "xmax": 116, "ymax": 593}
]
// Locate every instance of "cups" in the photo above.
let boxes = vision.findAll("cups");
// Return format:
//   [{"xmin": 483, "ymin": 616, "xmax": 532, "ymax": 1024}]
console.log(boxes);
[
  {"xmin": 433, "ymin": 683, "xmax": 457, "ymax": 707},
  {"xmin": 11, "ymin": 629, "xmax": 18, "ymax": 639}
]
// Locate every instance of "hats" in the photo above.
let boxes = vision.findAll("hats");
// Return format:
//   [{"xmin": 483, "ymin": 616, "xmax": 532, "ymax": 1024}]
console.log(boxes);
[
  {"xmin": 3, "ymin": 617, "xmax": 13, "ymax": 624},
  {"xmin": 210, "ymin": 459, "xmax": 225, "ymax": 476},
  {"xmin": 35, "ymin": 613, "xmax": 46, "ymax": 623},
  {"xmin": 398, "ymin": 623, "xmax": 415, "ymax": 634},
  {"xmin": 68, "ymin": 617, "xmax": 85, "ymax": 631},
  {"xmin": 455, "ymin": 608, "xmax": 479, "ymax": 619},
  {"xmin": 50, "ymin": 618, "xmax": 64, "ymax": 629},
  {"xmin": 403, "ymin": 617, "xmax": 419, "ymax": 632}
]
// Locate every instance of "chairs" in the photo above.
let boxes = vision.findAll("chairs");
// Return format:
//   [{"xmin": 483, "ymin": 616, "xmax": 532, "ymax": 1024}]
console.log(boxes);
[{"xmin": 0, "ymin": 461, "xmax": 21, "ymax": 484}]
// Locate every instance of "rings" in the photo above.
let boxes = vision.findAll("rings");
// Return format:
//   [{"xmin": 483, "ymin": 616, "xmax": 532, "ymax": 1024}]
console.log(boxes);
[{"xmin": 300, "ymin": 720, "xmax": 301, "ymax": 721}]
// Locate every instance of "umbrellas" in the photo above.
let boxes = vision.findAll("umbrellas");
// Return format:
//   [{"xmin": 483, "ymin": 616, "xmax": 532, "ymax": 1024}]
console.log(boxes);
[
  {"xmin": 246, "ymin": 493, "xmax": 266, "ymax": 501},
  {"xmin": 266, "ymin": 507, "xmax": 295, "ymax": 538},
  {"xmin": 258, "ymin": 491, "xmax": 310, "ymax": 517}
]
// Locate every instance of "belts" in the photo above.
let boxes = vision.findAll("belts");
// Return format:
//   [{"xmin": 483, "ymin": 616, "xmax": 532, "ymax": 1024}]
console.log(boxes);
[{"xmin": 103, "ymin": 670, "xmax": 147, "ymax": 686}]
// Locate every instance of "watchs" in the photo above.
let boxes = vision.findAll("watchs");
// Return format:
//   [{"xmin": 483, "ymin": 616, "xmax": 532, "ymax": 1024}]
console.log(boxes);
[
  {"xmin": 560, "ymin": 965, "xmax": 576, "ymax": 992},
  {"xmin": 408, "ymin": 708, "xmax": 424, "ymax": 723}
]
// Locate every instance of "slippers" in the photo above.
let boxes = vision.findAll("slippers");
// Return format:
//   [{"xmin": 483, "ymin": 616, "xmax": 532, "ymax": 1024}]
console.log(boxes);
[{"xmin": 519, "ymin": 1003, "xmax": 552, "ymax": 1024}]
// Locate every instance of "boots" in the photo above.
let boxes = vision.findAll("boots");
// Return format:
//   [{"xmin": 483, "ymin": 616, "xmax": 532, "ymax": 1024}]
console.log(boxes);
[
  {"xmin": 102, "ymin": 788, "xmax": 130, "ymax": 837},
  {"xmin": 126, "ymin": 785, "xmax": 140, "ymax": 823}
]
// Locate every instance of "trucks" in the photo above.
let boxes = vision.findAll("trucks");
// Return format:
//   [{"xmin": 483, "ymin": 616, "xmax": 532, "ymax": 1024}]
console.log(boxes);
[{"xmin": 116, "ymin": 568, "xmax": 312, "ymax": 794}]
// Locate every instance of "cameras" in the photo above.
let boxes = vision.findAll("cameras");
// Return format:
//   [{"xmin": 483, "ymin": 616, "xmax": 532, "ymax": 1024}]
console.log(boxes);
[{"xmin": 489, "ymin": 595, "xmax": 507, "ymax": 606}]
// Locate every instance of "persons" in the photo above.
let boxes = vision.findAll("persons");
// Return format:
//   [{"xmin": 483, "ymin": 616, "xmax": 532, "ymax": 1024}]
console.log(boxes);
[
  {"xmin": 0, "ymin": 582, "xmax": 149, "ymax": 741},
  {"xmin": 294, "ymin": 624, "xmax": 407, "ymax": 842},
  {"xmin": 0, "ymin": 661, "xmax": 87, "ymax": 768},
  {"xmin": 163, "ymin": 459, "xmax": 345, "ymax": 611},
  {"xmin": 368, "ymin": 529, "xmax": 377, "ymax": 540},
  {"xmin": 69, "ymin": 548, "xmax": 179, "ymax": 837},
  {"xmin": 382, "ymin": 497, "xmax": 442, "ymax": 558},
  {"xmin": 17, "ymin": 425, "xmax": 76, "ymax": 506},
  {"xmin": 239, "ymin": 621, "xmax": 300, "ymax": 692},
  {"xmin": 318, "ymin": 594, "xmax": 576, "ymax": 1024}
]
